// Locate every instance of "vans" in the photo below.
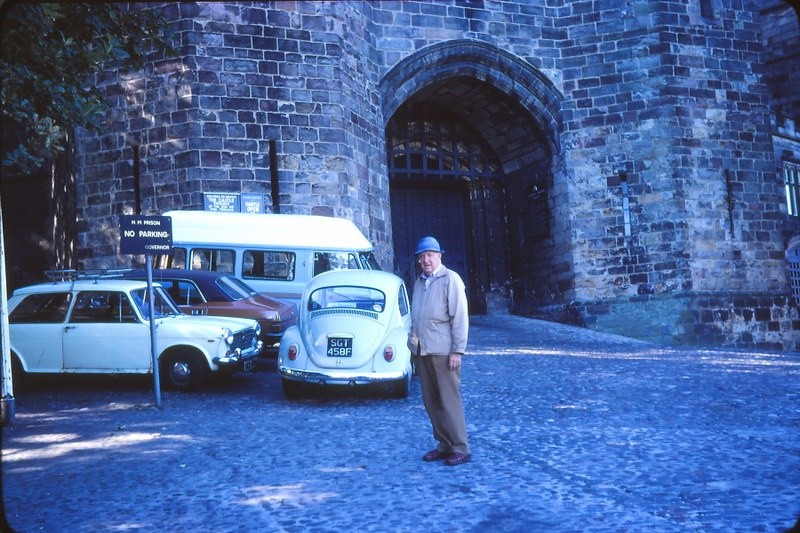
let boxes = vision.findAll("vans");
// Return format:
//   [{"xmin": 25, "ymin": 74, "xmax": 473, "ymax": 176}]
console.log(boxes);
[{"xmin": 152, "ymin": 209, "xmax": 384, "ymax": 311}]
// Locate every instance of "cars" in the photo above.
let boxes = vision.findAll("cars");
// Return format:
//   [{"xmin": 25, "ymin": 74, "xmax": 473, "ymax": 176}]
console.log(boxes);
[
  {"xmin": 277, "ymin": 268, "xmax": 413, "ymax": 399},
  {"xmin": 61, "ymin": 268, "xmax": 298, "ymax": 345}
]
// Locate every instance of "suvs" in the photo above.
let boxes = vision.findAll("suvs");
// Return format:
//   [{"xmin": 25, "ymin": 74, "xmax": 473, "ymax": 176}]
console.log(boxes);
[{"xmin": 4, "ymin": 279, "xmax": 265, "ymax": 392}]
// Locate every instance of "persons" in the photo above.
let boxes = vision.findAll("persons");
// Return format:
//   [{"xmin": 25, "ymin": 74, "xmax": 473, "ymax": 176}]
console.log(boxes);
[{"xmin": 406, "ymin": 237, "xmax": 472, "ymax": 465}]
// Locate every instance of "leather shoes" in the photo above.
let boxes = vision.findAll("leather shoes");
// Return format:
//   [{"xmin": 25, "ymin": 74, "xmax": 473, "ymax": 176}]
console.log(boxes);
[
  {"xmin": 445, "ymin": 452, "xmax": 471, "ymax": 464},
  {"xmin": 423, "ymin": 449, "xmax": 455, "ymax": 461}
]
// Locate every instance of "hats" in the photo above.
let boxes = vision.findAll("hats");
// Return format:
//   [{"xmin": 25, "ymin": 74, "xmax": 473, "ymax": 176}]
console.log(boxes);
[{"xmin": 415, "ymin": 237, "xmax": 444, "ymax": 254}]
